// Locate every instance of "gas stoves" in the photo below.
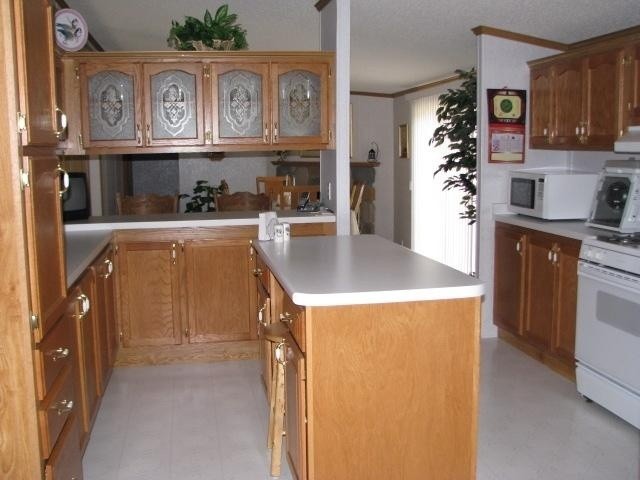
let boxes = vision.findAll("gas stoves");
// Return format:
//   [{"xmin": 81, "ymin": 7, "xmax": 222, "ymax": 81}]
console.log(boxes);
[{"xmin": 578, "ymin": 232, "xmax": 640, "ymax": 276}]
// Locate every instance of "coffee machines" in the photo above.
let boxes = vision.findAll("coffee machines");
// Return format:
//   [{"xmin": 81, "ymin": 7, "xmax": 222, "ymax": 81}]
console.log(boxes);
[{"xmin": 585, "ymin": 160, "xmax": 640, "ymax": 234}]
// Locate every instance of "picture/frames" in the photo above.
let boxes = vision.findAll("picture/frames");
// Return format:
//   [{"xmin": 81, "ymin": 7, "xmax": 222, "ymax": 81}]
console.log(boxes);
[{"xmin": 398, "ymin": 124, "xmax": 408, "ymax": 159}]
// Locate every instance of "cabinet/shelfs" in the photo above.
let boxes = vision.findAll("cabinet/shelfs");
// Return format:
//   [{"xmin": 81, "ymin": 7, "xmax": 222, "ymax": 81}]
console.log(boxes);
[
  {"xmin": 255, "ymin": 255, "xmax": 276, "ymax": 406},
  {"xmin": 118, "ymin": 238, "xmax": 260, "ymax": 345},
  {"xmin": 622, "ymin": 40, "xmax": 640, "ymax": 131},
  {"xmin": 279, "ymin": 292, "xmax": 309, "ymax": 480},
  {"xmin": 555, "ymin": 48, "xmax": 622, "ymax": 151},
  {"xmin": 77, "ymin": 60, "xmax": 206, "ymax": 146},
  {"xmin": 12, "ymin": 0, "xmax": 70, "ymax": 149},
  {"xmin": 528, "ymin": 236, "xmax": 577, "ymax": 368},
  {"xmin": 527, "ymin": 62, "xmax": 552, "ymax": 150},
  {"xmin": 208, "ymin": 60, "xmax": 327, "ymax": 145},
  {"xmin": 492, "ymin": 228, "xmax": 527, "ymax": 337},
  {"xmin": 73, "ymin": 244, "xmax": 117, "ymax": 439},
  {"xmin": 22, "ymin": 154, "xmax": 82, "ymax": 480}
]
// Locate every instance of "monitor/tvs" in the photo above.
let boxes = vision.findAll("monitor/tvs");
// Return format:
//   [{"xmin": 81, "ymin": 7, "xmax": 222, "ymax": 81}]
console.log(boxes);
[{"xmin": 60, "ymin": 172, "xmax": 92, "ymax": 222}]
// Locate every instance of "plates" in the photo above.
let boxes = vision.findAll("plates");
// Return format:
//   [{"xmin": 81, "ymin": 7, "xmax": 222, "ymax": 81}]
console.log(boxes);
[{"xmin": 54, "ymin": 9, "xmax": 88, "ymax": 51}]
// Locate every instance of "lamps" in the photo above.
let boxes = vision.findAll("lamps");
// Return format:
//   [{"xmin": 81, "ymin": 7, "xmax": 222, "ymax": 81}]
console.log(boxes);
[{"xmin": 367, "ymin": 141, "xmax": 379, "ymax": 164}]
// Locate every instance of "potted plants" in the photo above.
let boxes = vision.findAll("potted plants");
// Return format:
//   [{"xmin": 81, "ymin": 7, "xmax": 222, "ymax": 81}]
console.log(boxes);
[{"xmin": 167, "ymin": 4, "xmax": 248, "ymax": 51}]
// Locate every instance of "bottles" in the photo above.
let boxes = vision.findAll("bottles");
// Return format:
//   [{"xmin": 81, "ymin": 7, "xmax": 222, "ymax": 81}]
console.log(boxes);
[{"xmin": 280, "ymin": 174, "xmax": 298, "ymax": 210}]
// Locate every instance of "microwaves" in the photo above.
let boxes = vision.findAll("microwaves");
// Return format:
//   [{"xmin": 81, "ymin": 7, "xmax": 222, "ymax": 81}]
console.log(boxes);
[{"xmin": 507, "ymin": 167, "xmax": 600, "ymax": 223}]
